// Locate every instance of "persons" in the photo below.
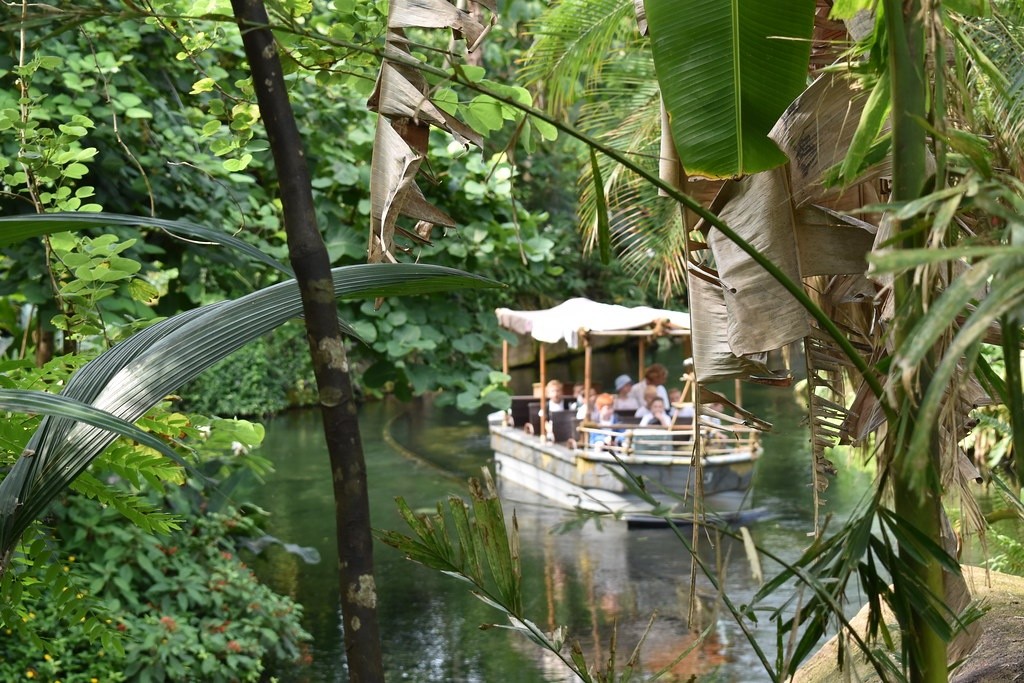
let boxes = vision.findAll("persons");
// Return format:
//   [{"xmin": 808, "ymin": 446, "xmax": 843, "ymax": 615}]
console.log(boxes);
[
  {"xmin": 615, "ymin": 375, "xmax": 638, "ymax": 411},
  {"xmin": 635, "ymin": 385, "xmax": 723, "ymax": 427},
  {"xmin": 573, "ymin": 382, "xmax": 586, "ymax": 396},
  {"xmin": 632, "ymin": 364, "xmax": 670, "ymax": 408},
  {"xmin": 538, "ymin": 380, "xmax": 573, "ymax": 440},
  {"xmin": 576, "ymin": 388, "xmax": 632, "ymax": 451}
]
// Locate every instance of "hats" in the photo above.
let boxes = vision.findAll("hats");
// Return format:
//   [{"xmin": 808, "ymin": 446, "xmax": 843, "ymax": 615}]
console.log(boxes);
[{"xmin": 615, "ymin": 375, "xmax": 632, "ymax": 391}]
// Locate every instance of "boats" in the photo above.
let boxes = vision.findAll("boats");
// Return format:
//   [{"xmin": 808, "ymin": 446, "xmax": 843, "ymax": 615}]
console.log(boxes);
[{"xmin": 486, "ymin": 294, "xmax": 772, "ymax": 526}]
